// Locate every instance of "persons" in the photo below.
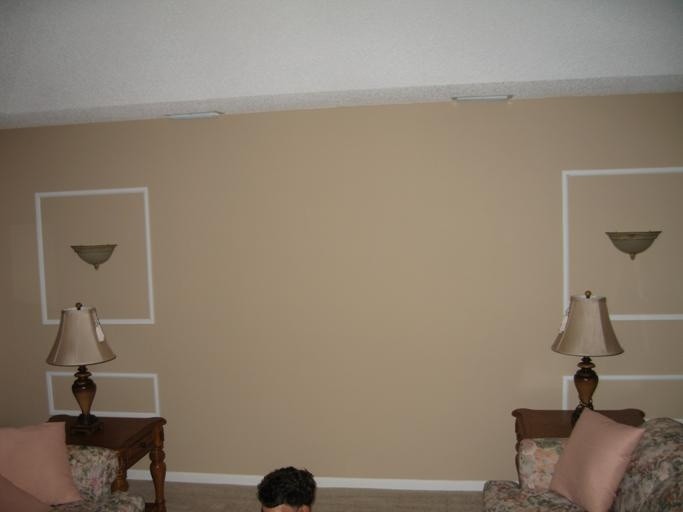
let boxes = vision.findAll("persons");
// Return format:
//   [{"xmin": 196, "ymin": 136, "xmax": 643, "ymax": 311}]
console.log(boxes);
[{"xmin": 257, "ymin": 467, "xmax": 316, "ymax": 512}]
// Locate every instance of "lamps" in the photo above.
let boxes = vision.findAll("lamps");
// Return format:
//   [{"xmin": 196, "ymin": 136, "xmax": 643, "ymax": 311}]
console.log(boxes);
[
  {"xmin": 66, "ymin": 242, "xmax": 118, "ymax": 272},
  {"xmin": 603, "ymin": 230, "xmax": 661, "ymax": 262},
  {"xmin": 550, "ymin": 291, "xmax": 623, "ymax": 429},
  {"xmin": 45, "ymin": 302, "xmax": 118, "ymax": 440}
]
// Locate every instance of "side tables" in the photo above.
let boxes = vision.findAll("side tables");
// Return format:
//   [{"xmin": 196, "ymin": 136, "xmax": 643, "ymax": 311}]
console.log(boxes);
[
  {"xmin": 44, "ymin": 413, "xmax": 168, "ymax": 511},
  {"xmin": 511, "ymin": 408, "xmax": 644, "ymax": 485}
]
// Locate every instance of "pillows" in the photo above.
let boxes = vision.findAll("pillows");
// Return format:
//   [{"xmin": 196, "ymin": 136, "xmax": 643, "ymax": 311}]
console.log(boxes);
[
  {"xmin": 0, "ymin": 423, "xmax": 83, "ymax": 506},
  {"xmin": 0, "ymin": 475, "xmax": 52, "ymax": 512},
  {"xmin": 548, "ymin": 408, "xmax": 645, "ymax": 512}
]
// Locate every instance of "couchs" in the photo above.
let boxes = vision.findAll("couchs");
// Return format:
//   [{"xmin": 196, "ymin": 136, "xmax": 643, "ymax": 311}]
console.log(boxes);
[
  {"xmin": 481, "ymin": 416, "xmax": 683, "ymax": 512},
  {"xmin": 0, "ymin": 446, "xmax": 144, "ymax": 512}
]
